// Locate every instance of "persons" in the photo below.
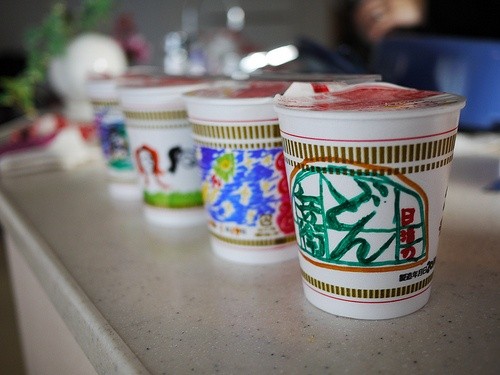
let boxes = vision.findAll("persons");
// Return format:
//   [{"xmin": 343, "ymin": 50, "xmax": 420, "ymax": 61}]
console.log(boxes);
[{"xmin": 356, "ymin": 1, "xmax": 500, "ymax": 49}]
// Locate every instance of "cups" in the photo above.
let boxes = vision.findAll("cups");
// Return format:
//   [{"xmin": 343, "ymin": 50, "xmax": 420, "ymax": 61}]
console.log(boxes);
[
  {"xmin": 120, "ymin": 82, "xmax": 210, "ymax": 226},
  {"xmin": 179, "ymin": 94, "xmax": 299, "ymax": 266},
  {"xmin": 63, "ymin": 76, "xmax": 153, "ymax": 181},
  {"xmin": 271, "ymin": 93, "xmax": 466, "ymax": 320}
]
